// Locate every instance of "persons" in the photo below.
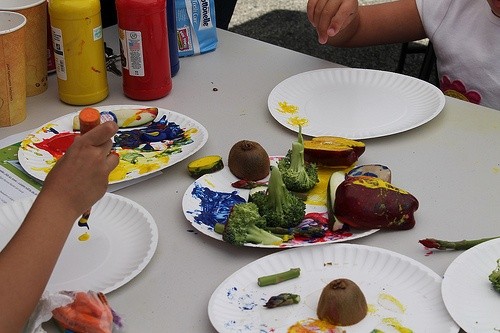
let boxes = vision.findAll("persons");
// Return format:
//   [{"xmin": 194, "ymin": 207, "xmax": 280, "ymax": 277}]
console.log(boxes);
[
  {"xmin": 306, "ymin": 0, "xmax": 500, "ymax": 111},
  {"xmin": 0, "ymin": 121, "xmax": 120, "ymax": 333}
]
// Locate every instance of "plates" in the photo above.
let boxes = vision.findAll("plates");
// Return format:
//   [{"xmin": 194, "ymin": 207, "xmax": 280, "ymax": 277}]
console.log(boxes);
[
  {"xmin": 17, "ymin": 104, "xmax": 208, "ymax": 188},
  {"xmin": 0, "ymin": 187, "xmax": 159, "ymax": 300},
  {"xmin": 181, "ymin": 155, "xmax": 388, "ymax": 249},
  {"xmin": 268, "ymin": 67, "xmax": 445, "ymax": 140},
  {"xmin": 206, "ymin": 241, "xmax": 462, "ymax": 333},
  {"xmin": 441, "ymin": 238, "xmax": 500, "ymax": 332}
]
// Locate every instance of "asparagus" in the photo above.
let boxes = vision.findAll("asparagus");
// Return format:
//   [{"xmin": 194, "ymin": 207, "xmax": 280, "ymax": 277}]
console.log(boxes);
[
  {"xmin": 215, "ymin": 223, "xmax": 325, "ymax": 238},
  {"xmin": 263, "ymin": 294, "xmax": 300, "ymax": 308},
  {"xmin": 419, "ymin": 236, "xmax": 500, "ymax": 249},
  {"xmin": 258, "ymin": 267, "xmax": 301, "ymax": 286}
]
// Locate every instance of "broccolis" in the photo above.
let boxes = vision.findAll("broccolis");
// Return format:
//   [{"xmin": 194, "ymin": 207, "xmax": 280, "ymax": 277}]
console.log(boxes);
[
  {"xmin": 489, "ymin": 258, "xmax": 500, "ymax": 294},
  {"xmin": 221, "ymin": 202, "xmax": 284, "ymax": 245},
  {"xmin": 248, "ymin": 165, "xmax": 305, "ymax": 226},
  {"xmin": 278, "ymin": 142, "xmax": 319, "ymax": 192}
]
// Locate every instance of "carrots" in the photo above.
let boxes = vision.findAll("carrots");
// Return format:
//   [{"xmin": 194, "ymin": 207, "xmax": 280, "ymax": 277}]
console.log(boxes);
[
  {"xmin": 78, "ymin": 108, "xmax": 101, "ymax": 226},
  {"xmin": 49, "ymin": 289, "xmax": 113, "ymax": 333}
]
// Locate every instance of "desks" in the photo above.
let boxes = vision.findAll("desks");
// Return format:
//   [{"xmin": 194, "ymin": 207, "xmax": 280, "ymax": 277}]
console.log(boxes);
[{"xmin": 0, "ymin": 23, "xmax": 500, "ymax": 333}]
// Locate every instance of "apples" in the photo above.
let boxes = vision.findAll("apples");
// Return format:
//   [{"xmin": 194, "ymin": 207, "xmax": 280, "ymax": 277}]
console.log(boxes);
[
  {"xmin": 303, "ymin": 137, "xmax": 365, "ymax": 166},
  {"xmin": 333, "ymin": 175, "xmax": 420, "ymax": 231}
]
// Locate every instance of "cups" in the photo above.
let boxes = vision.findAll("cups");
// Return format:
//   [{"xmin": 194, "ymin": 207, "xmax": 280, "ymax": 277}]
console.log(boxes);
[
  {"xmin": 0, "ymin": 8, "xmax": 30, "ymax": 127},
  {"xmin": 0, "ymin": 0, "xmax": 48, "ymax": 97}
]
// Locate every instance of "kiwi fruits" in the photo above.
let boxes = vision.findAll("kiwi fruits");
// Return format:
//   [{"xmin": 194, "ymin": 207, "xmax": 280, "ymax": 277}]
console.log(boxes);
[
  {"xmin": 228, "ymin": 140, "xmax": 270, "ymax": 181},
  {"xmin": 317, "ymin": 278, "xmax": 367, "ymax": 327}
]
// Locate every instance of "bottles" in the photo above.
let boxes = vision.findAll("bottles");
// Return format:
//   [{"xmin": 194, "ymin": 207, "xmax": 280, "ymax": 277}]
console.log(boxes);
[
  {"xmin": 165, "ymin": 0, "xmax": 188, "ymax": 76},
  {"xmin": 115, "ymin": 0, "xmax": 172, "ymax": 100},
  {"xmin": 47, "ymin": 0, "xmax": 109, "ymax": 106}
]
